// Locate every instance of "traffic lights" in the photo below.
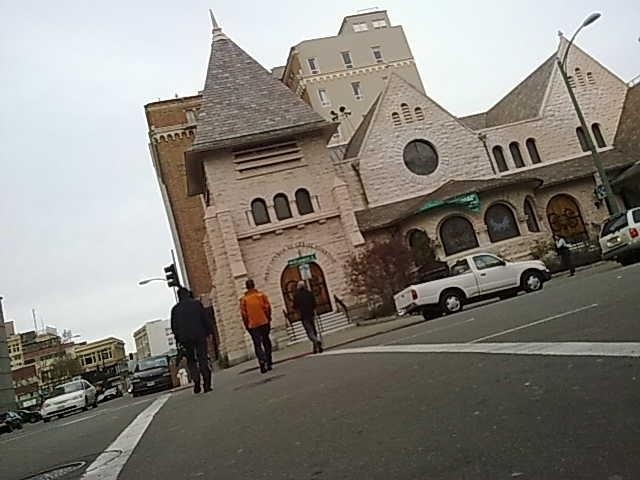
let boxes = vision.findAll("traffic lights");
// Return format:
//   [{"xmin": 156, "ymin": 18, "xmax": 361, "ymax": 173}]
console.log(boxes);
[{"xmin": 164, "ymin": 263, "xmax": 180, "ymax": 287}]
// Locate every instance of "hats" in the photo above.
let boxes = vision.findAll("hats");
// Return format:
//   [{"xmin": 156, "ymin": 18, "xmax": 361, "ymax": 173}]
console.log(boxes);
[{"xmin": 297, "ymin": 281, "xmax": 304, "ymax": 288}]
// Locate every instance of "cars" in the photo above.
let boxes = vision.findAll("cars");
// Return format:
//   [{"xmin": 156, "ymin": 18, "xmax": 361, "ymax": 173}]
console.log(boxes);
[{"xmin": 0, "ymin": 352, "xmax": 180, "ymax": 436}]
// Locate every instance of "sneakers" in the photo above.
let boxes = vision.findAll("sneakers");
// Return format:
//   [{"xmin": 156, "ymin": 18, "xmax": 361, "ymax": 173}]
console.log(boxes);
[
  {"xmin": 313, "ymin": 340, "xmax": 323, "ymax": 354},
  {"xmin": 204, "ymin": 385, "xmax": 213, "ymax": 393},
  {"xmin": 194, "ymin": 385, "xmax": 202, "ymax": 393},
  {"xmin": 261, "ymin": 367, "xmax": 272, "ymax": 373}
]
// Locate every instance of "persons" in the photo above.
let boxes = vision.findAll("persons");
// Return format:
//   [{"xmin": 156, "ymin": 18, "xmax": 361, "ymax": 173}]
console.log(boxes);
[
  {"xmin": 171, "ymin": 287, "xmax": 214, "ymax": 394},
  {"xmin": 239, "ymin": 279, "xmax": 273, "ymax": 373},
  {"xmin": 292, "ymin": 280, "xmax": 323, "ymax": 354},
  {"xmin": 553, "ymin": 233, "xmax": 576, "ymax": 277}
]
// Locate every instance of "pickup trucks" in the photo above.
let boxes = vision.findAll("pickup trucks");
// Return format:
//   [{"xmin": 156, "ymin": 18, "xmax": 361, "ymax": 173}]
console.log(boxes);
[{"xmin": 393, "ymin": 252, "xmax": 550, "ymax": 321}]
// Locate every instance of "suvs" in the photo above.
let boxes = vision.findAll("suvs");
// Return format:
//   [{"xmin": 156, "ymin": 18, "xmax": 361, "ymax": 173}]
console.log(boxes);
[{"xmin": 598, "ymin": 205, "xmax": 640, "ymax": 267}]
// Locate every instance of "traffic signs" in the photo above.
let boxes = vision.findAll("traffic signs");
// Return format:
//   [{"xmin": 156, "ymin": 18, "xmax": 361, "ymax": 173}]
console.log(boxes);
[{"xmin": 287, "ymin": 253, "xmax": 317, "ymax": 269}]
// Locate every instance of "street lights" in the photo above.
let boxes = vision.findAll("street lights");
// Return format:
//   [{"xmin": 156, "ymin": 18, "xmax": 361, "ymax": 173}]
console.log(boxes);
[
  {"xmin": 555, "ymin": 11, "xmax": 622, "ymax": 214},
  {"xmin": 138, "ymin": 278, "xmax": 178, "ymax": 303}
]
